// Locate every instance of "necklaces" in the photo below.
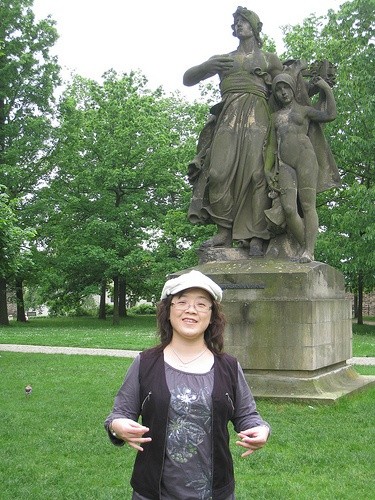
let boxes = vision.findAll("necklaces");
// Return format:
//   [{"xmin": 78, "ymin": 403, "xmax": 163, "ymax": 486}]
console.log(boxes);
[{"xmin": 170, "ymin": 344, "xmax": 207, "ymax": 368}]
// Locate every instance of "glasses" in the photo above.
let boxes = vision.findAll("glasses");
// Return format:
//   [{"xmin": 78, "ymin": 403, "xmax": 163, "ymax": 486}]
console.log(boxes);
[{"xmin": 170, "ymin": 299, "xmax": 213, "ymax": 312}]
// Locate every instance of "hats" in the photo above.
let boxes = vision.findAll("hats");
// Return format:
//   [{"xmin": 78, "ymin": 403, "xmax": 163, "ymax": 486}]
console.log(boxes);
[{"xmin": 160, "ymin": 269, "xmax": 223, "ymax": 306}]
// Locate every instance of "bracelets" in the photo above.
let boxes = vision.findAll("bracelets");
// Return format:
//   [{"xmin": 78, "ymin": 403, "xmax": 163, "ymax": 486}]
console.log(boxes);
[{"xmin": 110, "ymin": 422, "xmax": 121, "ymax": 440}]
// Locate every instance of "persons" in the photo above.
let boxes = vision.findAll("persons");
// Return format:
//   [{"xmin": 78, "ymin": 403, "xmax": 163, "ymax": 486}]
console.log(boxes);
[
  {"xmin": 104, "ymin": 272, "xmax": 270, "ymax": 500},
  {"xmin": 183, "ymin": 6, "xmax": 284, "ymax": 258},
  {"xmin": 263, "ymin": 73, "xmax": 337, "ymax": 265}
]
